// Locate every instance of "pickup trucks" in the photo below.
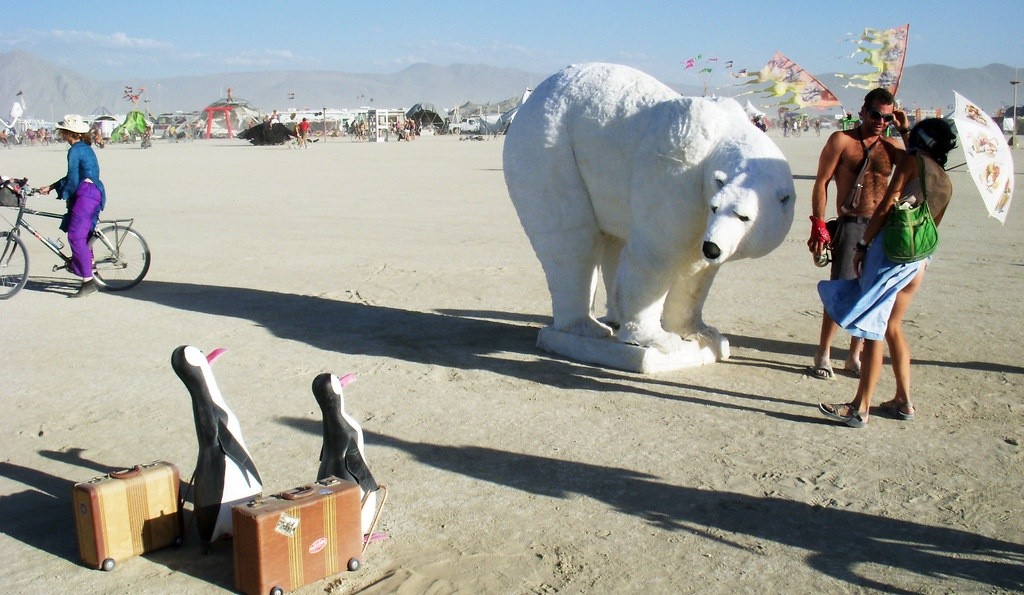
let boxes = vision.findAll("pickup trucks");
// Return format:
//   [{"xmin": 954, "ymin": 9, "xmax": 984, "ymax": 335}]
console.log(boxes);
[{"xmin": 448, "ymin": 118, "xmax": 481, "ymax": 135}]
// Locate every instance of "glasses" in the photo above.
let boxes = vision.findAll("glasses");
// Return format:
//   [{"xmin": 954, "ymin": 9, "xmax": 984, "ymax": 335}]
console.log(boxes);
[
  {"xmin": 864, "ymin": 104, "xmax": 893, "ymax": 121},
  {"xmin": 60, "ymin": 129, "xmax": 66, "ymax": 132}
]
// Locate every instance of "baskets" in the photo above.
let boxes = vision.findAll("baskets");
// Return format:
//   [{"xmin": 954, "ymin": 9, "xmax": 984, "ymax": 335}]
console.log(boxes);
[{"xmin": 0, "ymin": 180, "xmax": 29, "ymax": 207}]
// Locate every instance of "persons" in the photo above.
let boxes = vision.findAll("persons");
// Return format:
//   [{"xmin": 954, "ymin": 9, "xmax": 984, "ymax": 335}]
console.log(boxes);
[
  {"xmin": 819, "ymin": 115, "xmax": 957, "ymax": 427},
  {"xmin": 295, "ymin": 117, "xmax": 312, "ymax": 150},
  {"xmin": 782, "ymin": 117, "xmax": 821, "ymax": 137},
  {"xmin": 93, "ymin": 128, "xmax": 106, "ymax": 149},
  {"xmin": 35, "ymin": 114, "xmax": 107, "ymax": 300},
  {"xmin": 0, "ymin": 130, "xmax": 11, "ymax": 149},
  {"xmin": 123, "ymin": 125, "xmax": 153, "ymax": 146},
  {"xmin": 389, "ymin": 118, "xmax": 416, "ymax": 142},
  {"xmin": 346, "ymin": 120, "xmax": 366, "ymax": 142},
  {"xmin": 248, "ymin": 122, "xmax": 254, "ymax": 129},
  {"xmin": 807, "ymin": 89, "xmax": 910, "ymax": 379},
  {"xmin": 752, "ymin": 117, "xmax": 766, "ymax": 131},
  {"xmin": 226, "ymin": 89, "xmax": 234, "ymax": 102},
  {"xmin": 168, "ymin": 129, "xmax": 178, "ymax": 143},
  {"xmin": 270, "ymin": 110, "xmax": 280, "ymax": 130},
  {"xmin": 25, "ymin": 127, "xmax": 51, "ymax": 146}
]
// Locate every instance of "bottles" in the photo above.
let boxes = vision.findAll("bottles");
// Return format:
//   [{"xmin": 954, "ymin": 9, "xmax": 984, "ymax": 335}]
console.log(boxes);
[{"xmin": 813, "ymin": 241, "xmax": 829, "ymax": 267}]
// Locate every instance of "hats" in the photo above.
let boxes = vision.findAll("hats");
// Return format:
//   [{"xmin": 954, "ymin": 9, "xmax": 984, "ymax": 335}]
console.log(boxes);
[{"xmin": 55, "ymin": 115, "xmax": 90, "ymax": 133}]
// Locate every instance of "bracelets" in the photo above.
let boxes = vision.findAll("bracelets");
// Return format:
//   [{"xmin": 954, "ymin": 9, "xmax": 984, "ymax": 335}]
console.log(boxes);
[{"xmin": 853, "ymin": 238, "xmax": 868, "ymax": 253}]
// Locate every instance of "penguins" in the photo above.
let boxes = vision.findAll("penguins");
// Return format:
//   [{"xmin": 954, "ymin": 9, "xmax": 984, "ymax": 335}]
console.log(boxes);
[
  {"xmin": 170, "ymin": 345, "xmax": 262, "ymax": 553},
  {"xmin": 311, "ymin": 372, "xmax": 388, "ymax": 544}
]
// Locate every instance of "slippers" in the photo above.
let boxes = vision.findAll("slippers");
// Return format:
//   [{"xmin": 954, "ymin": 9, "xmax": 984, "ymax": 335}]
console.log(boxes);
[
  {"xmin": 807, "ymin": 365, "xmax": 836, "ymax": 380},
  {"xmin": 844, "ymin": 366, "xmax": 861, "ymax": 378}
]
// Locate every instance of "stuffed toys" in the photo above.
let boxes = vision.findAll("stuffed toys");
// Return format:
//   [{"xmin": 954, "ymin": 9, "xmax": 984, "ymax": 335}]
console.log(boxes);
[
  {"xmin": 170, "ymin": 344, "xmax": 264, "ymax": 557},
  {"xmin": 311, "ymin": 371, "xmax": 388, "ymax": 545}
]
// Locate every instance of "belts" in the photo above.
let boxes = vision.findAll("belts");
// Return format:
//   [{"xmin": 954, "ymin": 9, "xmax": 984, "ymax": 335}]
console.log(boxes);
[{"xmin": 838, "ymin": 216, "xmax": 871, "ymax": 224}]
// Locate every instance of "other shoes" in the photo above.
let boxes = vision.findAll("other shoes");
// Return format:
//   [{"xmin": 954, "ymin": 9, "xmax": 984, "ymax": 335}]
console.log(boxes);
[
  {"xmin": 68, "ymin": 279, "xmax": 98, "ymax": 298},
  {"xmin": 94, "ymin": 272, "xmax": 103, "ymax": 282}
]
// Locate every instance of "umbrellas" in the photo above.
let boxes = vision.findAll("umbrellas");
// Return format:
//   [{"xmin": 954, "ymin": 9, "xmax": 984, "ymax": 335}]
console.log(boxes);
[{"xmin": 944, "ymin": 89, "xmax": 1015, "ymax": 228}]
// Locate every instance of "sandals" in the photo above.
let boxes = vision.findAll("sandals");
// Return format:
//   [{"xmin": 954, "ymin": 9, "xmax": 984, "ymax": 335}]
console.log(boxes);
[
  {"xmin": 879, "ymin": 400, "xmax": 916, "ymax": 420},
  {"xmin": 819, "ymin": 402, "xmax": 864, "ymax": 428}
]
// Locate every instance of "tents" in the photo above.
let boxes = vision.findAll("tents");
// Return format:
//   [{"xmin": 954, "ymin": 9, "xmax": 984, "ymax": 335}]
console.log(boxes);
[{"xmin": 743, "ymin": 100, "xmax": 766, "ymax": 118}]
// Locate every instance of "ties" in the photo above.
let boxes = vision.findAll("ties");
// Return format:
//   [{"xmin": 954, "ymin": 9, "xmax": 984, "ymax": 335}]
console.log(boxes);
[{"xmin": 841, "ymin": 126, "xmax": 880, "ymax": 210}]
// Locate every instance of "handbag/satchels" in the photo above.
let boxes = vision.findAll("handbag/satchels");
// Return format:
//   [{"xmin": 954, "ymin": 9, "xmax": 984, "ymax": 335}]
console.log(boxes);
[{"xmin": 882, "ymin": 152, "xmax": 939, "ymax": 264}]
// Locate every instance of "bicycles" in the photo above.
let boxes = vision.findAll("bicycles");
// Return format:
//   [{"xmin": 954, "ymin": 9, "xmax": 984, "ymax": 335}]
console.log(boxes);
[{"xmin": 0, "ymin": 177, "xmax": 151, "ymax": 301}]
